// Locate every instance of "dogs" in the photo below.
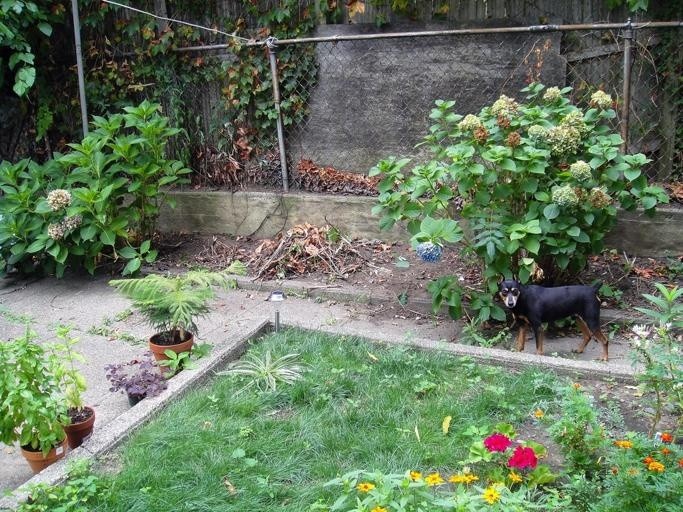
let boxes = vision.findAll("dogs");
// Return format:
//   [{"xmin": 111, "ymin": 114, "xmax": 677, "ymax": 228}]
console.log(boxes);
[{"xmin": 496, "ymin": 273, "xmax": 608, "ymax": 361}]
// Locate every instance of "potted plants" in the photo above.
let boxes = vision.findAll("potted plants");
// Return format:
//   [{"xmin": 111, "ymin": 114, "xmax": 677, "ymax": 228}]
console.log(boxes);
[
  {"xmin": 42, "ymin": 323, "xmax": 95, "ymax": 450},
  {"xmin": 108, "ymin": 260, "xmax": 247, "ymax": 371},
  {"xmin": 104, "ymin": 349, "xmax": 169, "ymax": 406},
  {"xmin": 2, "ymin": 321, "xmax": 71, "ymax": 472}
]
